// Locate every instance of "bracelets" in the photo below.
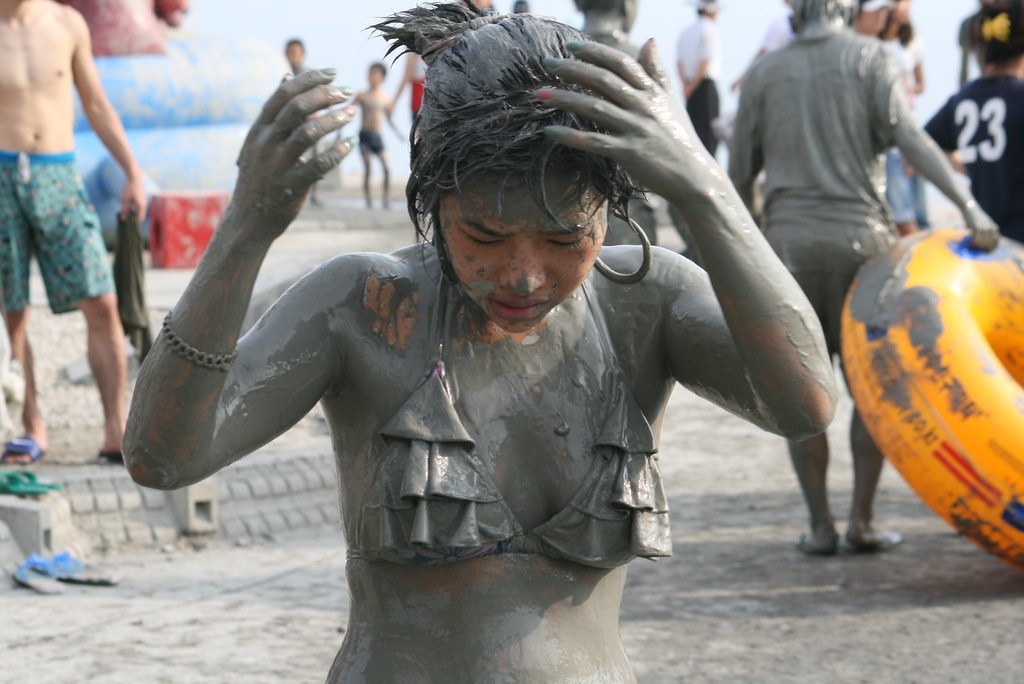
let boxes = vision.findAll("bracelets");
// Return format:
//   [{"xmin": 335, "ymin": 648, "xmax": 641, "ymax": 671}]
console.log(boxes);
[{"xmin": 162, "ymin": 311, "xmax": 237, "ymax": 369}]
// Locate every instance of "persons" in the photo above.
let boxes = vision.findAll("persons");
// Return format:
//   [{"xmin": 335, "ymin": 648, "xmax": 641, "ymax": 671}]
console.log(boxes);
[
  {"xmin": 285, "ymin": 0, "xmax": 1024, "ymax": 244},
  {"xmin": 0, "ymin": 0, "xmax": 147, "ymax": 465},
  {"xmin": 119, "ymin": 0, "xmax": 839, "ymax": 684},
  {"xmin": 728, "ymin": 0, "xmax": 1001, "ymax": 555}
]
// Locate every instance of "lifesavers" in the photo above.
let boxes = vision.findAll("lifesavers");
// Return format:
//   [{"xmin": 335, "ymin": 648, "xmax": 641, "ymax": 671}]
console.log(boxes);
[{"xmin": 839, "ymin": 211, "xmax": 1024, "ymax": 572}]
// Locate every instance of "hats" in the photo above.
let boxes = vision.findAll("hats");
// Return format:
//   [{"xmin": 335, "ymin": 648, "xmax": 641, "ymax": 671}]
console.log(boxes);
[{"xmin": 855, "ymin": 0, "xmax": 897, "ymax": 13}]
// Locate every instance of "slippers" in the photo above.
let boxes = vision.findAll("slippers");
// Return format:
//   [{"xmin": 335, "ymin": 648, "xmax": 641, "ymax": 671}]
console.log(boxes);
[
  {"xmin": 0, "ymin": 469, "xmax": 62, "ymax": 494},
  {"xmin": 1, "ymin": 436, "xmax": 47, "ymax": 464},
  {"xmin": 98, "ymin": 449, "xmax": 123, "ymax": 465},
  {"xmin": 49, "ymin": 551, "xmax": 119, "ymax": 585},
  {"xmin": 12, "ymin": 555, "xmax": 64, "ymax": 595}
]
